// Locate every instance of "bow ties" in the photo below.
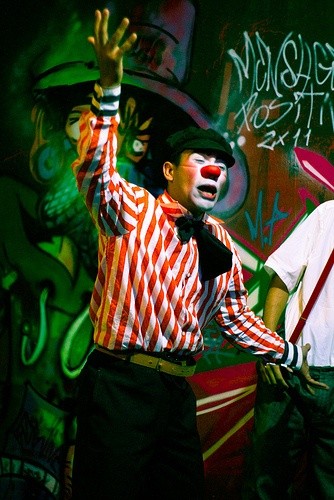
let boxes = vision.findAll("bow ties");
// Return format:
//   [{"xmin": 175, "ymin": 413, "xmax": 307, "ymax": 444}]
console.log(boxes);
[{"xmin": 173, "ymin": 211, "xmax": 233, "ymax": 281}]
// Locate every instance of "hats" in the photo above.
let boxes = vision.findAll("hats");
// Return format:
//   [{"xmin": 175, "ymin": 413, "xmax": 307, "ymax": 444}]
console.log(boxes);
[{"xmin": 165, "ymin": 127, "xmax": 237, "ymax": 168}]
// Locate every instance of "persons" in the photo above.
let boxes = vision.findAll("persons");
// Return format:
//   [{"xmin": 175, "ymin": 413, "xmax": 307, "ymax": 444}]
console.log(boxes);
[
  {"xmin": 242, "ymin": 198, "xmax": 334, "ymax": 500},
  {"xmin": 69, "ymin": 10, "xmax": 329, "ymax": 500}
]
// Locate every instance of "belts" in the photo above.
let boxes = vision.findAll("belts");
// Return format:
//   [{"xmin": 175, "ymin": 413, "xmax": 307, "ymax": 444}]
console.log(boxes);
[{"xmin": 93, "ymin": 338, "xmax": 198, "ymax": 378}]
